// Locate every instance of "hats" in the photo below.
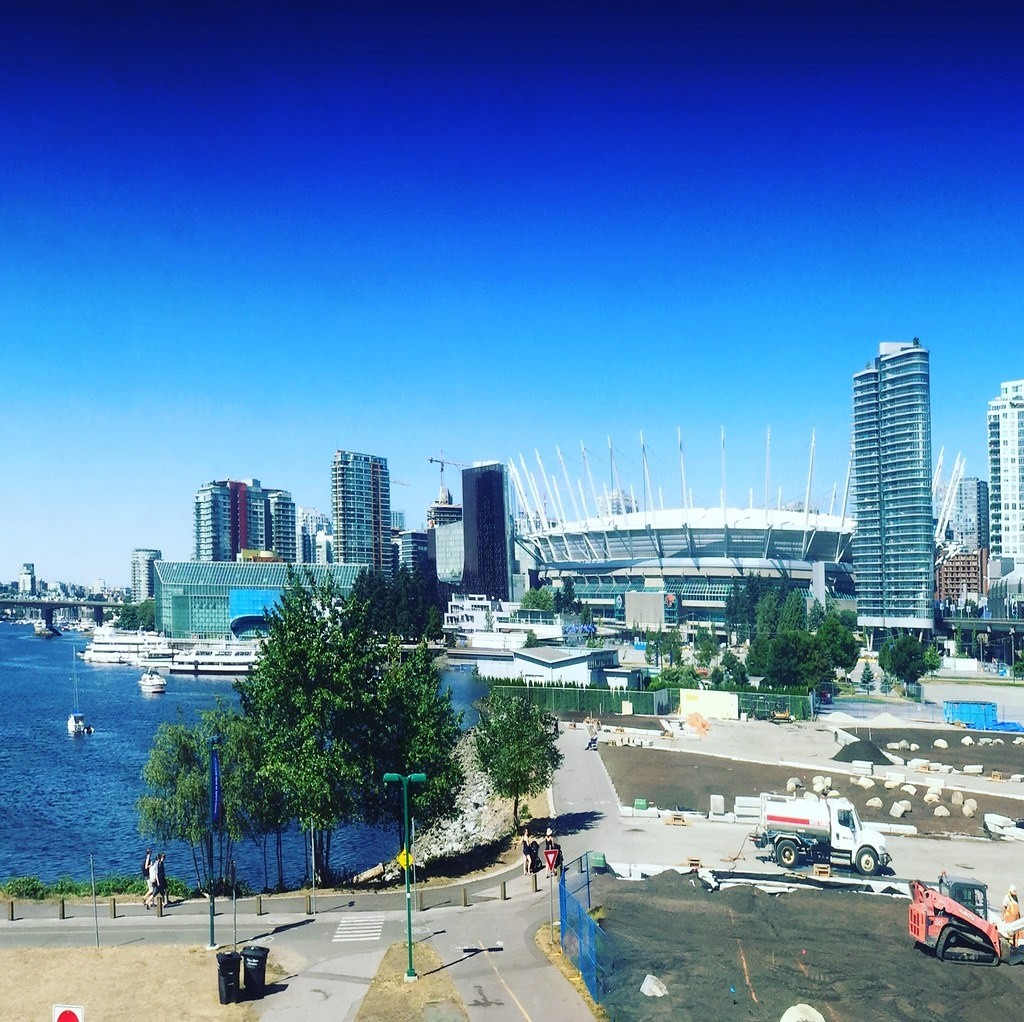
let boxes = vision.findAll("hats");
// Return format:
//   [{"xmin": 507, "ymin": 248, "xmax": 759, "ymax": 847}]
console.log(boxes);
[{"xmin": 546, "ymin": 828, "xmax": 552, "ymax": 836}]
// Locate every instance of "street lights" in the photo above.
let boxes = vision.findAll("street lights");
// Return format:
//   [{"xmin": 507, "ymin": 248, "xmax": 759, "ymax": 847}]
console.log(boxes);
[{"xmin": 382, "ymin": 773, "xmax": 427, "ymax": 981}]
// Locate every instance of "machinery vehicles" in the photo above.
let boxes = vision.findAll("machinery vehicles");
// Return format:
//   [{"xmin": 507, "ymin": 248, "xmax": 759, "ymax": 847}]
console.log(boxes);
[
  {"xmin": 747, "ymin": 792, "xmax": 892, "ymax": 878},
  {"xmin": 907, "ymin": 868, "xmax": 1024, "ymax": 965},
  {"xmin": 748, "ymin": 694, "xmax": 790, "ymax": 723}
]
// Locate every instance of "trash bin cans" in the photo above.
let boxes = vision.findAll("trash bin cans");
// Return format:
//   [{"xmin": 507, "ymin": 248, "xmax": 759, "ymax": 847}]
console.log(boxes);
[
  {"xmin": 216, "ymin": 950, "xmax": 242, "ymax": 1005},
  {"xmin": 239, "ymin": 946, "xmax": 270, "ymax": 993}
]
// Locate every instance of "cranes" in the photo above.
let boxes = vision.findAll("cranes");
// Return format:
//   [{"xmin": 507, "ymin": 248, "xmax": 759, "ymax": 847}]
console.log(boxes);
[{"xmin": 428, "ymin": 447, "xmax": 471, "ymax": 505}]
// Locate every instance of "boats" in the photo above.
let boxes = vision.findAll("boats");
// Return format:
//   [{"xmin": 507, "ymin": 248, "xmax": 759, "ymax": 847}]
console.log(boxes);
[
  {"xmin": 138, "ymin": 666, "xmax": 168, "ymax": 694},
  {"xmin": 10, "ymin": 618, "xmax": 97, "ymax": 634},
  {"xmin": 67, "ymin": 711, "xmax": 94, "ymax": 735}
]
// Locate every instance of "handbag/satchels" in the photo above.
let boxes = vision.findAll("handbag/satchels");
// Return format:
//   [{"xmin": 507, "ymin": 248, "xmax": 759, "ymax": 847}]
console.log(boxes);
[{"xmin": 141, "ymin": 863, "xmax": 149, "ymax": 876}]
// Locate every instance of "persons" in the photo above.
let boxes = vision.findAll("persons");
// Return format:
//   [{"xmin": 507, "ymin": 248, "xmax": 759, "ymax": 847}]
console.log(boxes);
[
  {"xmin": 584, "ymin": 731, "xmax": 598, "ymax": 750},
  {"xmin": 521, "ymin": 828, "xmax": 531, "ymax": 876},
  {"xmin": 141, "ymin": 849, "xmax": 169, "ymax": 911},
  {"xmin": 1001, "ymin": 884, "xmax": 1019, "ymax": 948},
  {"xmin": 937, "ymin": 868, "xmax": 947, "ymax": 884},
  {"xmin": 544, "ymin": 827, "xmax": 555, "ymax": 879}
]
